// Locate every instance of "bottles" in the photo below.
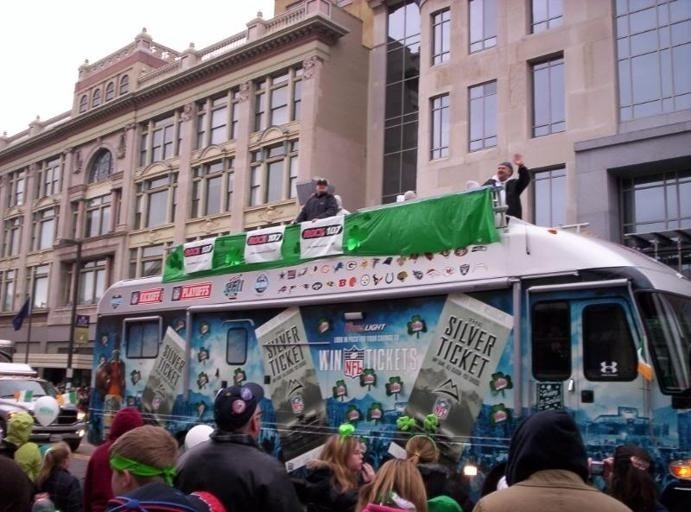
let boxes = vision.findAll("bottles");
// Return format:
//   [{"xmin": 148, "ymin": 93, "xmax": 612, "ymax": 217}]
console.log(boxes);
[{"xmin": 104, "ymin": 341, "xmax": 129, "ymax": 443}]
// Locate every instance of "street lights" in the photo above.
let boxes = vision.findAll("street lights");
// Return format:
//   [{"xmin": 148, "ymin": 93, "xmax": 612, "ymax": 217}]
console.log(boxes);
[{"xmin": 51, "ymin": 236, "xmax": 83, "ymax": 394}]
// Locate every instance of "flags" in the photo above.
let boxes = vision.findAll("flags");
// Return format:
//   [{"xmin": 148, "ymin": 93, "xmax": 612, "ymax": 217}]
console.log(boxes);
[{"xmin": 12, "ymin": 297, "xmax": 30, "ymax": 331}]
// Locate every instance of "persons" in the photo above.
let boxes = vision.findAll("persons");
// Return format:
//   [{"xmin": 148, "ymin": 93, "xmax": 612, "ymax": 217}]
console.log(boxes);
[
  {"xmin": 473, "ymin": 411, "xmax": 633, "ymax": 512},
  {"xmin": 56, "ymin": 382, "xmax": 94, "ymax": 412},
  {"xmin": 587, "ymin": 445, "xmax": 691, "ymax": 512},
  {"xmin": 0, "ymin": 409, "xmax": 84, "ymax": 512},
  {"xmin": 335, "ymin": 195, "xmax": 358, "ymax": 216},
  {"xmin": 97, "ymin": 354, "xmax": 107, "ymax": 369},
  {"xmin": 172, "ymin": 382, "xmax": 308, "ymax": 512},
  {"xmin": 106, "ymin": 349, "xmax": 126, "ymax": 400},
  {"xmin": 83, "ymin": 407, "xmax": 216, "ymax": 512},
  {"xmin": 482, "ymin": 151, "xmax": 530, "ymax": 222},
  {"xmin": 403, "ymin": 191, "xmax": 416, "ymax": 202},
  {"xmin": 289, "ymin": 433, "xmax": 510, "ymax": 512},
  {"xmin": 293, "ymin": 176, "xmax": 339, "ymax": 222}
]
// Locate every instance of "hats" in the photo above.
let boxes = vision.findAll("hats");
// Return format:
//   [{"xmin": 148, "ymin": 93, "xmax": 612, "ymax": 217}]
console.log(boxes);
[
  {"xmin": 213, "ymin": 382, "xmax": 264, "ymax": 429},
  {"xmin": 497, "ymin": 161, "xmax": 514, "ymax": 174},
  {"xmin": 315, "ymin": 179, "xmax": 328, "ymax": 186}
]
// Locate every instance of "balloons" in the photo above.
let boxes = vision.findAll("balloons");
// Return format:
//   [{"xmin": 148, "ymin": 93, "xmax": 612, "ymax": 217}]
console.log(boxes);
[{"xmin": 34, "ymin": 395, "xmax": 59, "ymax": 426}]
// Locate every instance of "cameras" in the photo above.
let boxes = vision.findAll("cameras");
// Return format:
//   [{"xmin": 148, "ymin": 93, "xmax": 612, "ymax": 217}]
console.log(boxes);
[{"xmin": 592, "ymin": 461, "xmax": 605, "ymax": 476}]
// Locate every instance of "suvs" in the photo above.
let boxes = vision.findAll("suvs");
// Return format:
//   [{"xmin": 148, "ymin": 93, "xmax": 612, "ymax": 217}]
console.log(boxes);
[{"xmin": 0, "ymin": 361, "xmax": 87, "ymax": 454}]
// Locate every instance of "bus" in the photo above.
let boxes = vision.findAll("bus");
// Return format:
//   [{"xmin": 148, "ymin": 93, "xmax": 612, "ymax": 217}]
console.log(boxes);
[{"xmin": 88, "ymin": 182, "xmax": 691, "ymax": 509}]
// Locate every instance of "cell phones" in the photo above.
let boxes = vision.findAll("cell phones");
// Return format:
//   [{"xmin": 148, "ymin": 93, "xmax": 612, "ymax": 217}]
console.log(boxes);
[{"xmin": 463, "ymin": 466, "xmax": 478, "ymax": 475}]
